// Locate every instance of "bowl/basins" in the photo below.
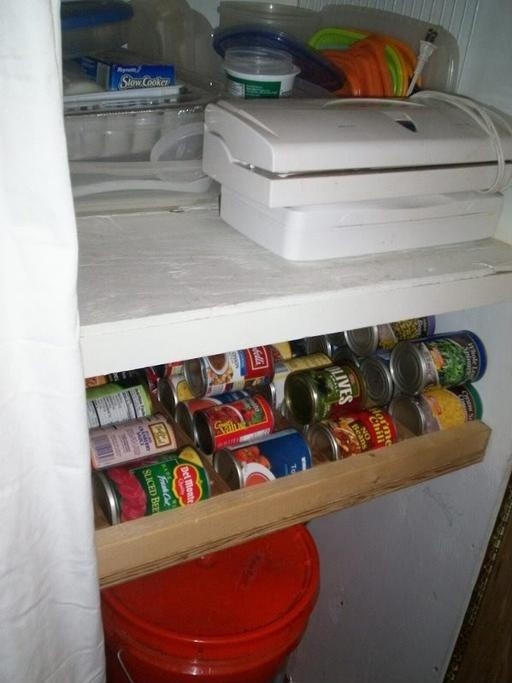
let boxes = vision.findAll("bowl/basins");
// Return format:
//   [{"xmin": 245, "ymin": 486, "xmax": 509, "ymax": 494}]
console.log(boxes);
[{"xmin": 217, "ymin": 0, "xmax": 320, "ymax": 43}]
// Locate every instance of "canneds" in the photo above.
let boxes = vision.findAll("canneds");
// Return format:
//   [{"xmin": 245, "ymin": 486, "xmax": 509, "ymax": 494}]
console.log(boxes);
[{"xmin": 85, "ymin": 317, "xmax": 487, "ymax": 526}]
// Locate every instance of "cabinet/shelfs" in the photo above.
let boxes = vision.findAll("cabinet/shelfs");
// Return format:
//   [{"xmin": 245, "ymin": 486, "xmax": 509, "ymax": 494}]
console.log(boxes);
[{"xmin": 58, "ymin": 0, "xmax": 512, "ymax": 681}]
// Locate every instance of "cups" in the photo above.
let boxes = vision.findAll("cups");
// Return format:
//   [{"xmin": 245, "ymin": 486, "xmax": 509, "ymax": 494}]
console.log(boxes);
[{"xmin": 222, "ymin": 62, "xmax": 302, "ymax": 99}]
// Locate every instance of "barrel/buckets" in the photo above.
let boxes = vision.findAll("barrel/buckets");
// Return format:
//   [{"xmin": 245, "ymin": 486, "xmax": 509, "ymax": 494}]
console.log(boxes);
[{"xmin": 100, "ymin": 523, "xmax": 321, "ymax": 683}]
